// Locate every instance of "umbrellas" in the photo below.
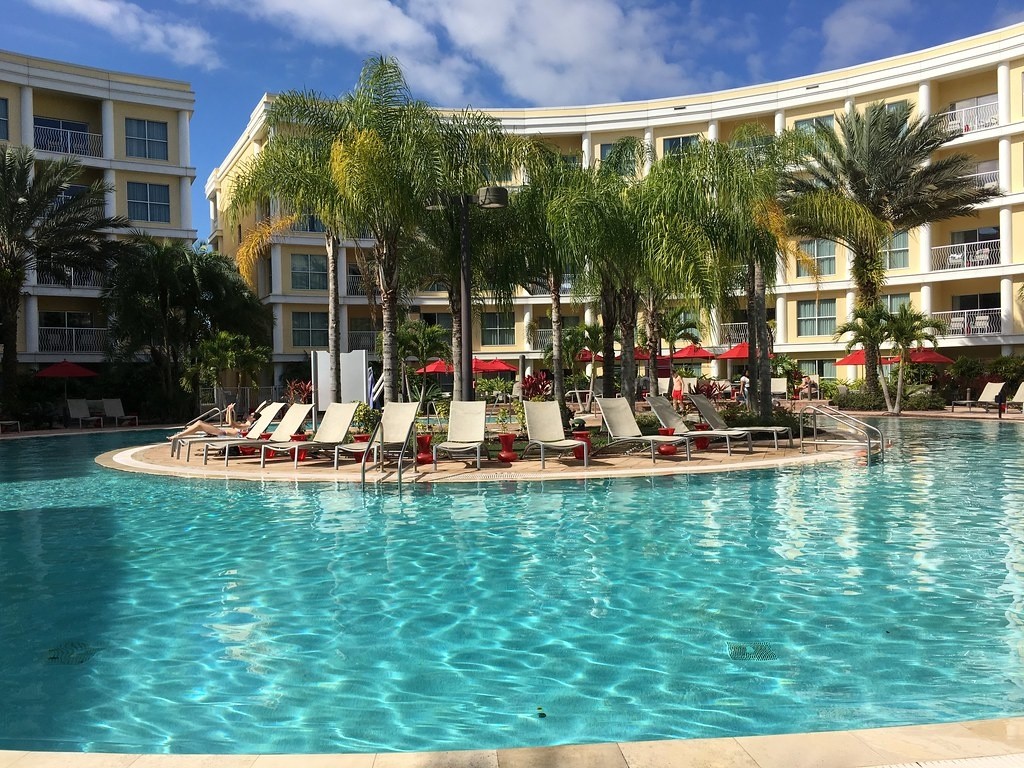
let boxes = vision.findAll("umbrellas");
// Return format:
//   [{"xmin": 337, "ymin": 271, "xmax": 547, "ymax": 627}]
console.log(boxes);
[
  {"xmin": 662, "ymin": 344, "xmax": 716, "ymax": 368},
  {"xmin": 717, "ymin": 341, "xmax": 777, "ymax": 370},
  {"xmin": 473, "ymin": 356, "xmax": 505, "ymax": 381},
  {"xmin": 487, "ymin": 357, "xmax": 519, "ymax": 376},
  {"xmin": 35, "ymin": 357, "xmax": 101, "ymax": 409},
  {"xmin": 573, "ymin": 349, "xmax": 604, "ymax": 362},
  {"xmin": 833, "ymin": 346, "xmax": 894, "ymax": 384},
  {"xmin": 614, "ymin": 346, "xmax": 665, "ymax": 378},
  {"xmin": 890, "ymin": 344, "xmax": 956, "ymax": 386},
  {"xmin": 416, "ymin": 358, "xmax": 454, "ymax": 373}
]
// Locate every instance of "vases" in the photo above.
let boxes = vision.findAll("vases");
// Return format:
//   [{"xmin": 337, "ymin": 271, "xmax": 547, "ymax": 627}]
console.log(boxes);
[
  {"xmin": 792, "ymin": 389, "xmax": 799, "ymax": 400},
  {"xmin": 290, "ymin": 435, "xmax": 308, "ymax": 461},
  {"xmin": 497, "ymin": 434, "xmax": 518, "ymax": 462},
  {"xmin": 352, "ymin": 435, "xmax": 371, "ymax": 463},
  {"xmin": 657, "ymin": 427, "xmax": 676, "ymax": 456},
  {"xmin": 259, "ymin": 433, "xmax": 275, "ymax": 459},
  {"xmin": 693, "ymin": 424, "xmax": 711, "ymax": 451},
  {"xmin": 572, "ymin": 431, "xmax": 592, "ymax": 460},
  {"xmin": 416, "ymin": 435, "xmax": 433, "ymax": 465},
  {"xmin": 731, "ymin": 389, "xmax": 737, "ymax": 399},
  {"xmin": 1001, "ymin": 403, "xmax": 1008, "ymax": 412}
]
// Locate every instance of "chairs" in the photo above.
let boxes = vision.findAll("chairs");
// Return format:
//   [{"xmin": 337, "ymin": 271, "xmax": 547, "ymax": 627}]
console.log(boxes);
[
  {"xmin": 771, "ymin": 378, "xmax": 787, "ymax": 400},
  {"xmin": 951, "ymin": 382, "xmax": 1006, "ymax": 412},
  {"xmin": 972, "ymin": 248, "xmax": 990, "ymax": 266},
  {"xmin": 646, "ymin": 377, "xmax": 670, "ymax": 398},
  {"xmin": 170, "ymin": 401, "xmax": 287, "ymax": 462},
  {"xmin": 683, "ymin": 377, "xmax": 698, "ymax": 396},
  {"xmin": 986, "ymin": 381, "xmax": 1024, "ymax": 414},
  {"xmin": 102, "ymin": 400, "xmax": 137, "ymax": 427},
  {"xmin": 985, "ymin": 181, "xmax": 997, "ymax": 189},
  {"xmin": 432, "ymin": 400, "xmax": 491, "ymax": 471},
  {"xmin": 522, "ymin": 400, "xmax": 589, "ymax": 469},
  {"xmin": 799, "ymin": 375, "xmax": 820, "ymax": 400},
  {"xmin": 204, "ymin": 403, "xmax": 317, "ymax": 466},
  {"xmin": 65, "ymin": 398, "xmax": 103, "ymax": 431},
  {"xmin": 970, "ymin": 316, "xmax": 990, "ymax": 334},
  {"xmin": 589, "ymin": 396, "xmax": 690, "ymax": 461},
  {"xmin": 643, "ymin": 396, "xmax": 753, "ymax": 456},
  {"xmin": 593, "ymin": 379, "xmax": 603, "ymax": 398},
  {"xmin": 0, "ymin": 421, "xmax": 20, "ymax": 434},
  {"xmin": 948, "ymin": 120, "xmax": 962, "ymax": 133},
  {"xmin": 947, "ymin": 254, "xmax": 964, "ymax": 268},
  {"xmin": 261, "ymin": 400, "xmax": 362, "ymax": 469},
  {"xmin": 979, "ymin": 114, "xmax": 999, "ymax": 129},
  {"xmin": 334, "ymin": 402, "xmax": 422, "ymax": 471},
  {"xmin": 688, "ymin": 394, "xmax": 794, "ymax": 450},
  {"xmin": 709, "ymin": 380, "xmax": 733, "ymax": 396},
  {"xmin": 945, "ymin": 316, "xmax": 965, "ymax": 334}
]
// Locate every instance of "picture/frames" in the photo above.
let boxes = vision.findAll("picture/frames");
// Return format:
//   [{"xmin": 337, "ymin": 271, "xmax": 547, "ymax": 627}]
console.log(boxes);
[{"xmin": 239, "ymin": 432, "xmax": 256, "ymax": 454}]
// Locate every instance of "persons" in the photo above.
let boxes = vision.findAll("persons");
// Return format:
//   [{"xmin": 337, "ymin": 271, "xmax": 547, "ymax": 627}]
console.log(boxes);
[
  {"xmin": 671, "ymin": 370, "xmax": 685, "ymax": 413},
  {"xmin": 166, "ymin": 403, "xmax": 262, "ymax": 442},
  {"xmin": 739, "ymin": 370, "xmax": 752, "ymax": 413},
  {"xmin": 473, "ymin": 379, "xmax": 477, "ymax": 401},
  {"xmin": 636, "ymin": 376, "xmax": 645, "ymax": 402},
  {"xmin": 798, "ymin": 375, "xmax": 818, "ymax": 393}
]
